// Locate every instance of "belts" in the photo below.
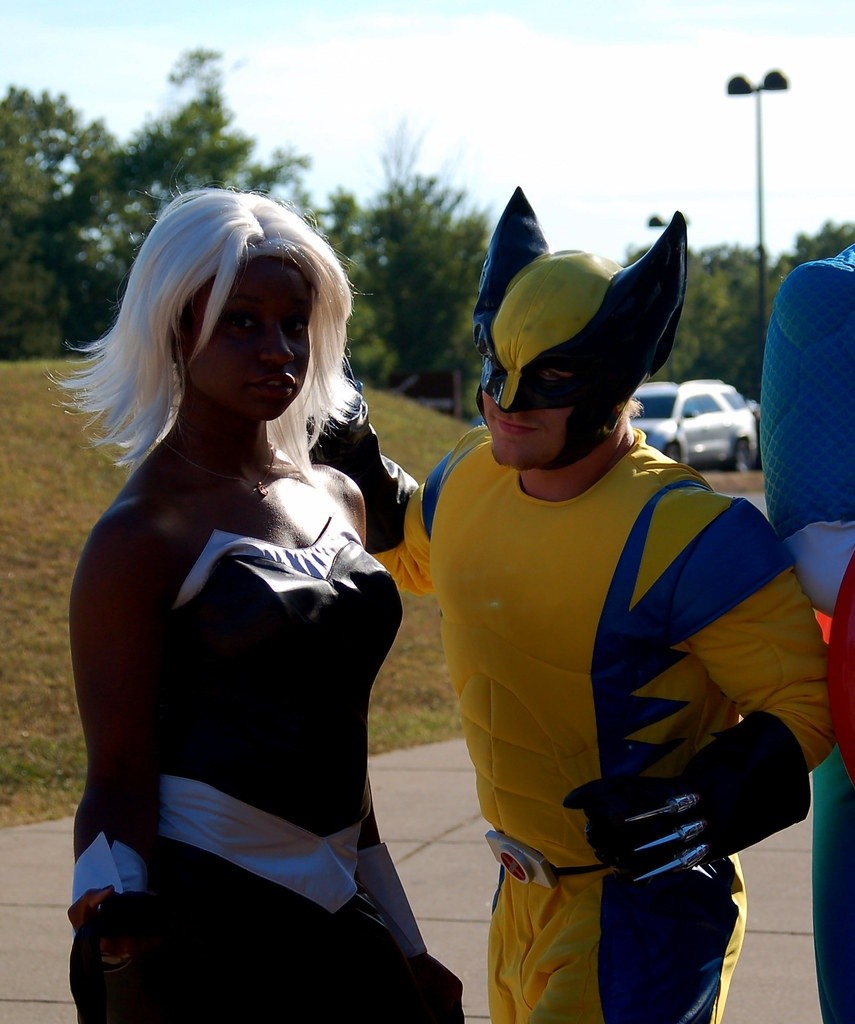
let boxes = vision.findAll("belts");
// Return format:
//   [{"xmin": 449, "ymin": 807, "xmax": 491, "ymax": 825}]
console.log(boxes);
[{"xmin": 485, "ymin": 830, "xmax": 610, "ymax": 889}]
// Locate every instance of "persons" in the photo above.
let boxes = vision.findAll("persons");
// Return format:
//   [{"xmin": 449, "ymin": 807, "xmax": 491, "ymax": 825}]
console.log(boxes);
[
  {"xmin": 761, "ymin": 230, "xmax": 855, "ymax": 1024},
  {"xmin": 305, "ymin": 184, "xmax": 834, "ymax": 1024},
  {"xmin": 44, "ymin": 190, "xmax": 468, "ymax": 1022}
]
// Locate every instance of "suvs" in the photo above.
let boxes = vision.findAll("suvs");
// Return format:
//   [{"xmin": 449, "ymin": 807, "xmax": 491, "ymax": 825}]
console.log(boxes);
[{"xmin": 628, "ymin": 377, "xmax": 759, "ymax": 476}]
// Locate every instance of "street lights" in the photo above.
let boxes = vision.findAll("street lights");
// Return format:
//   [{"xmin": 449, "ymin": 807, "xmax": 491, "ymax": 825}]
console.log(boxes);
[{"xmin": 727, "ymin": 71, "xmax": 789, "ymax": 359}]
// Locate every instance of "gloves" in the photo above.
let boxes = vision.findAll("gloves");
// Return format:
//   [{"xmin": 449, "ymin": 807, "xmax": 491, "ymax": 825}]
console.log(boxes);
[{"xmin": 561, "ymin": 708, "xmax": 811, "ymax": 887}]
